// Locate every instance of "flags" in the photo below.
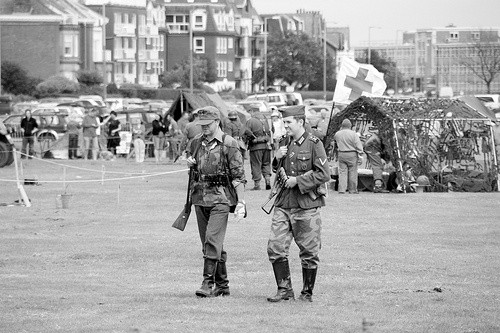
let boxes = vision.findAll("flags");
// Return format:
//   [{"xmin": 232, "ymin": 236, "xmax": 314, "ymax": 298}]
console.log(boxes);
[{"xmin": 334, "ymin": 56, "xmax": 386, "ymax": 105}]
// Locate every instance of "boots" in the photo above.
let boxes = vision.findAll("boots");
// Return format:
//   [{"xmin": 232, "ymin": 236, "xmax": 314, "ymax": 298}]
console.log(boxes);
[
  {"xmin": 196, "ymin": 258, "xmax": 219, "ymax": 297},
  {"xmin": 301, "ymin": 267, "xmax": 317, "ymax": 302},
  {"xmin": 266, "ymin": 180, "xmax": 271, "ymax": 189},
  {"xmin": 267, "ymin": 261, "xmax": 294, "ymax": 302},
  {"xmin": 212, "ymin": 262, "xmax": 230, "ymax": 296},
  {"xmin": 250, "ymin": 180, "xmax": 260, "ymax": 190}
]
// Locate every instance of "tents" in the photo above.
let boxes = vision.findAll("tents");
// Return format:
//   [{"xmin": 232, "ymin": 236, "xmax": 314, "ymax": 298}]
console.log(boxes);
[
  {"xmin": 146, "ymin": 93, "xmax": 229, "ymax": 139},
  {"xmin": 326, "ymin": 96, "xmax": 500, "ymax": 193}
]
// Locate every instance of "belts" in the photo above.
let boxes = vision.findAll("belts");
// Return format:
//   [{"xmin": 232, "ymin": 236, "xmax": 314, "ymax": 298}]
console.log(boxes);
[{"xmin": 338, "ymin": 150, "xmax": 357, "ymax": 152}]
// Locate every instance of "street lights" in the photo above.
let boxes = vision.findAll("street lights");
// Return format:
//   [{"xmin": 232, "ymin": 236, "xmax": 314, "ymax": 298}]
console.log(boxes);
[
  {"xmin": 265, "ymin": 16, "xmax": 274, "ymax": 94},
  {"xmin": 323, "ymin": 20, "xmax": 338, "ymax": 105},
  {"xmin": 102, "ymin": 0, "xmax": 112, "ymax": 100},
  {"xmin": 188, "ymin": 8, "xmax": 199, "ymax": 97},
  {"xmin": 394, "ymin": 29, "xmax": 405, "ymax": 101},
  {"xmin": 367, "ymin": 23, "xmax": 376, "ymax": 66}
]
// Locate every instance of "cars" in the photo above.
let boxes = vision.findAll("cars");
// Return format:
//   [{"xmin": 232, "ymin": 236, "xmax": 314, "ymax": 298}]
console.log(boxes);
[
  {"xmin": 474, "ymin": 93, "xmax": 500, "ymax": 120},
  {"xmin": 0, "ymin": 96, "xmax": 104, "ymax": 167},
  {"xmin": 101, "ymin": 107, "xmax": 162, "ymax": 139},
  {"xmin": 74, "ymin": 94, "xmax": 173, "ymax": 130},
  {"xmin": 224, "ymin": 92, "xmax": 350, "ymax": 122}
]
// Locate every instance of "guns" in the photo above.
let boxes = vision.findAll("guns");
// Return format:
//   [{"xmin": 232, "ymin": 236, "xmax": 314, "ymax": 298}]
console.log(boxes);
[
  {"xmin": 261, "ymin": 193, "xmax": 278, "ymax": 214},
  {"xmin": 172, "ymin": 197, "xmax": 193, "ymax": 232}
]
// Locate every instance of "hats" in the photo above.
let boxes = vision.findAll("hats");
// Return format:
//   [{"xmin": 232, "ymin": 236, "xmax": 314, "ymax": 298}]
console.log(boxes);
[
  {"xmin": 196, "ymin": 106, "xmax": 221, "ymax": 126},
  {"xmin": 247, "ymin": 104, "xmax": 259, "ymax": 112},
  {"xmin": 282, "ymin": 106, "xmax": 305, "ymax": 118},
  {"xmin": 271, "ymin": 113, "xmax": 280, "ymax": 117},
  {"xmin": 228, "ymin": 111, "xmax": 238, "ymax": 118}
]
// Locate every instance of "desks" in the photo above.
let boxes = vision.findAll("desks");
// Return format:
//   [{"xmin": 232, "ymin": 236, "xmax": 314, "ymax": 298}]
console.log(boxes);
[{"xmin": 358, "ymin": 174, "xmax": 390, "ymax": 192}]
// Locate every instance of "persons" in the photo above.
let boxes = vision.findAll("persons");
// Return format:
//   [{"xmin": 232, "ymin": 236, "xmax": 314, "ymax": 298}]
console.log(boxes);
[
  {"xmin": 67, "ymin": 109, "xmax": 122, "ymax": 160},
  {"xmin": 363, "ymin": 128, "xmax": 407, "ymax": 193},
  {"xmin": 21, "ymin": 109, "xmax": 39, "ymax": 160},
  {"xmin": 133, "ymin": 119, "xmax": 147, "ymax": 162},
  {"xmin": 186, "ymin": 106, "xmax": 248, "ymax": 297},
  {"xmin": 152, "ymin": 111, "xmax": 203, "ymax": 160},
  {"xmin": 229, "ymin": 104, "xmax": 329, "ymax": 191},
  {"xmin": 335, "ymin": 119, "xmax": 363, "ymax": 194},
  {"xmin": 267, "ymin": 106, "xmax": 331, "ymax": 302}
]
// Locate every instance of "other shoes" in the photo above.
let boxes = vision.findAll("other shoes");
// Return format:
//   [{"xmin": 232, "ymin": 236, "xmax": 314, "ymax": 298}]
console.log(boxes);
[{"xmin": 373, "ymin": 185, "xmax": 390, "ymax": 193}]
